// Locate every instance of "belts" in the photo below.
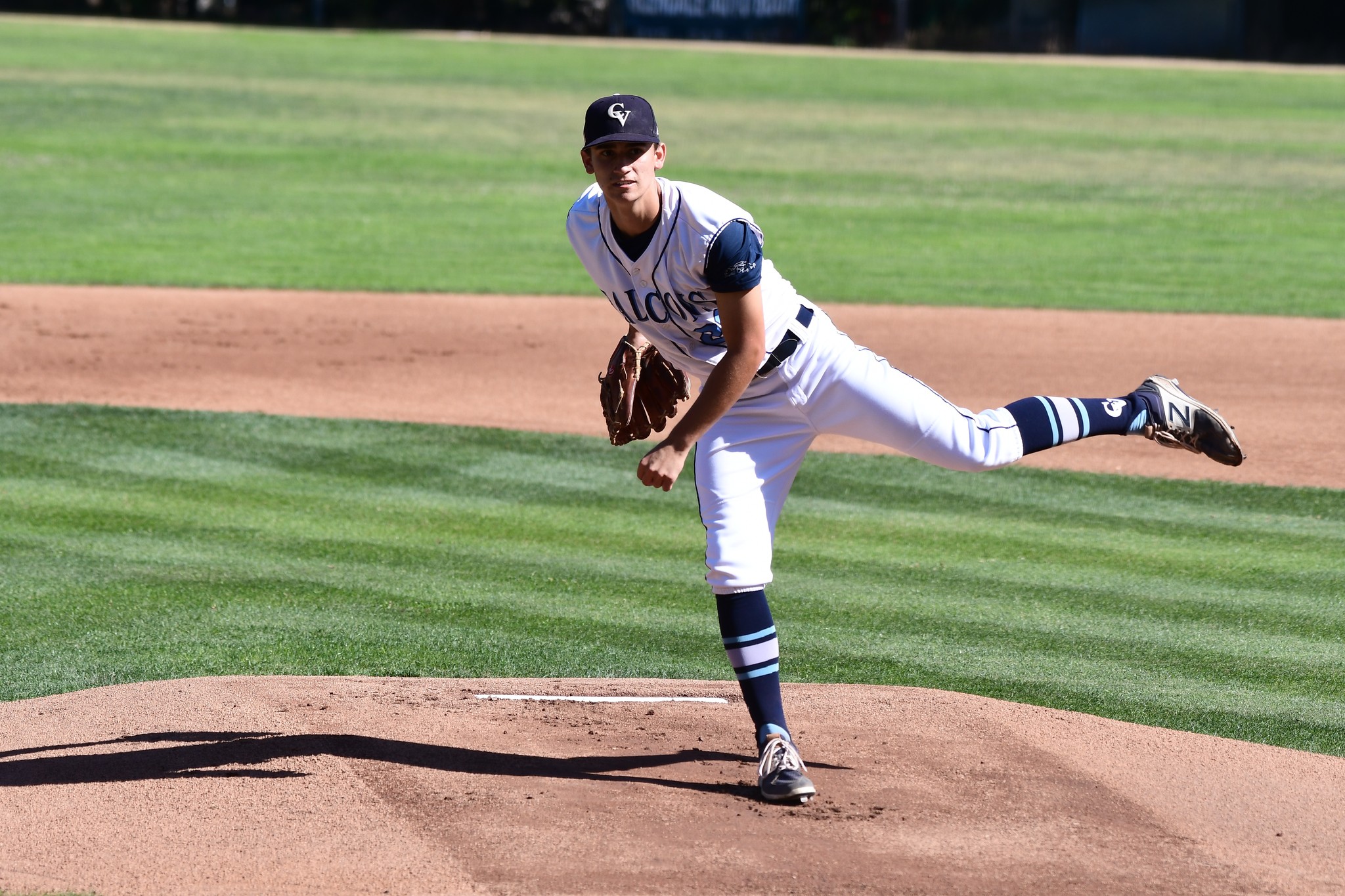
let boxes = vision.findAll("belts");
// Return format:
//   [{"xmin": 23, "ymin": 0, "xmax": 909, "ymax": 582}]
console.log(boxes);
[{"xmin": 753, "ymin": 304, "xmax": 813, "ymax": 380}]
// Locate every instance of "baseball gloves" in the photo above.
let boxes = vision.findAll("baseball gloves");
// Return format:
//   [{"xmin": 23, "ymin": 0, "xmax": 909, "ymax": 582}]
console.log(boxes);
[{"xmin": 598, "ymin": 332, "xmax": 691, "ymax": 447}]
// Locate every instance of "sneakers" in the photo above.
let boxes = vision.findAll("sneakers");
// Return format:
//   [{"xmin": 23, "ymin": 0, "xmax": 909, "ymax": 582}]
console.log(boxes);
[
  {"xmin": 758, "ymin": 732, "xmax": 815, "ymax": 803},
  {"xmin": 1130, "ymin": 375, "xmax": 1243, "ymax": 467}
]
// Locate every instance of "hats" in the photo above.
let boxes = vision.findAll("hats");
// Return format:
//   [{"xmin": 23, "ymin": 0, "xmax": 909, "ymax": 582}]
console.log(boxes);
[{"xmin": 583, "ymin": 94, "xmax": 661, "ymax": 152}]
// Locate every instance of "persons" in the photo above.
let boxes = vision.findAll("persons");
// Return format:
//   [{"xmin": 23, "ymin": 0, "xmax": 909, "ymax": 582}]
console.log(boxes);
[{"xmin": 564, "ymin": 93, "xmax": 1248, "ymax": 803}]
